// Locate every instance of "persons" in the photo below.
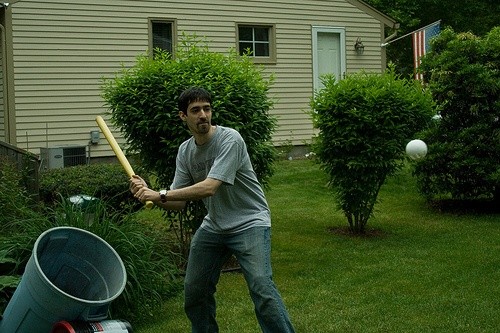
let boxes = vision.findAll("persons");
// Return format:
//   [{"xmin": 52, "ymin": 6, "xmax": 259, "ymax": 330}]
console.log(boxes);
[{"xmin": 128, "ymin": 87, "xmax": 295, "ymax": 333}]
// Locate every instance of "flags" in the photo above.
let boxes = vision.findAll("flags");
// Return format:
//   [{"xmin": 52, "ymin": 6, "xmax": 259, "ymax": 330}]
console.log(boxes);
[{"xmin": 412, "ymin": 19, "xmax": 441, "ymax": 89}]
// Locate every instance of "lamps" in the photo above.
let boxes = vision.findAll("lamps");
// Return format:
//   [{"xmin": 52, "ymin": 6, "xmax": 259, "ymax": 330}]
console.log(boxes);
[{"xmin": 354, "ymin": 35, "xmax": 364, "ymax": 56}]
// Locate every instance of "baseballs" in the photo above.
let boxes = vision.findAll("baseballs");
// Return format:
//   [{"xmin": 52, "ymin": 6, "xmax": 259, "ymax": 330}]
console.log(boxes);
[{"xmin": 405, "ymin": 139, "xmax": 428, "ymax": 160}]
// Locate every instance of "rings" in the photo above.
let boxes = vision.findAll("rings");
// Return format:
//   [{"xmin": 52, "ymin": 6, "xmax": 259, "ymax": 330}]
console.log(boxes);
[{"xmin": 138, "ymin": 196, "xmax": 142, "ymax": 199}]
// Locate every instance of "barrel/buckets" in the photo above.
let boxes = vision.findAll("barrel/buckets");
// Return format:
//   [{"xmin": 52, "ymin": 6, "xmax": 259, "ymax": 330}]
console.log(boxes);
[
  {"xmin": 0, "ymin": 225, "xmax": 128, "ymax": 333},
  {"xmin": 52, "ymin": 319, "xmax": 133, "ymax": 333}
]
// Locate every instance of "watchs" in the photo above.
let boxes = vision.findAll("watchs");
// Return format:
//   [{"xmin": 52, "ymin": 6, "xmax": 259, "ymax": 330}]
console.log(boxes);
[{"xmin": 159, "ymin": 189, "xmax": 167, "ymax": 203}]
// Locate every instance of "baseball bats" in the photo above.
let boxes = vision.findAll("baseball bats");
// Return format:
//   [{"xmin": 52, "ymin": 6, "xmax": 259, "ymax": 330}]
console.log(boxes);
[{"xmin": 95, "ymin": 116, "xmax": 154, "ymax": 209}]
